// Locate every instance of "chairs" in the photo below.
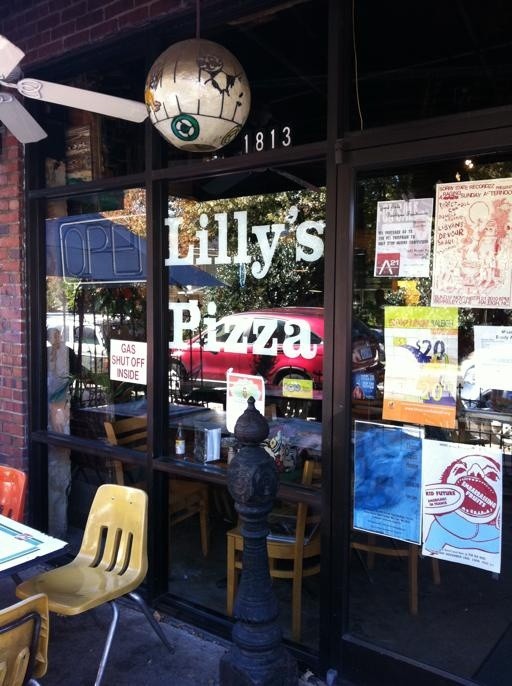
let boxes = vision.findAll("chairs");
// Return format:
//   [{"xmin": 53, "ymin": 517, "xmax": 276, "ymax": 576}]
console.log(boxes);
[
  {"xmin": 1, "ymin": 591, "xmax": 50, "ymax": 686},
  {"xmin": 104, "ymin": 414, "xmax": 209, "ymax": 555},
  {"xmin": 3, "ymin": 464, "xmax": 27, "ymax": 521},
  {"xmin": 353, "ymin": 540, "xmax": 439, "ymax": 616},
  {"xmin": 225, "ymin": 461, "xmax": 324, "ymax": 641},
  {"xmin": 16, "ymin": 483, "xmax": 175, "ymax": 686}
]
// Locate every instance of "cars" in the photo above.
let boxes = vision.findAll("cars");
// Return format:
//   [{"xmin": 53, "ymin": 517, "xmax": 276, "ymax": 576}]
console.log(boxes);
[
  {"xmin": 169, "ymin": 307, "xmax": 385, "ymax": 396},
  {"xmin": 46, "ymin": 313, "xmax": 146, "ymax": 375}
]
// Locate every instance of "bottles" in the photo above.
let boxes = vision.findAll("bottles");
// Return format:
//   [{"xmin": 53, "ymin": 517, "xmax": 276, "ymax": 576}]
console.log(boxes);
[{"xmin": 175, "ymin": 419, "xmax": 185, "ymax": 459}]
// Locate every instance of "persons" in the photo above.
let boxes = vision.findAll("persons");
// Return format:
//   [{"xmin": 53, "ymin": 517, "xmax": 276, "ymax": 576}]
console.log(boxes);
[{"xmin": 46, "ymin": 328, "xmax": 82, "ymax": 377}]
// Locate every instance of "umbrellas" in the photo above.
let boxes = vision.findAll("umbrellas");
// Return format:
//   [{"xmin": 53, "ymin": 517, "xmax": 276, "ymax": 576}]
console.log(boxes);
[{"xmin": 44, "ymin": 213, "xmax": 227, "ymax": 377}]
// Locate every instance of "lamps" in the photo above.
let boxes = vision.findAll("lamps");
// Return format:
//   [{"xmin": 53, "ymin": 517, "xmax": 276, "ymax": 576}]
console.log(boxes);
[{"xmin": 144, "ymin": 0, "xmax": 251, "ymax": 152}]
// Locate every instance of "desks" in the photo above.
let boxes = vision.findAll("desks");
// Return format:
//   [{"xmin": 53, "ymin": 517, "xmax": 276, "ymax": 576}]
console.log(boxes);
[
  {"xmin": 1, "ymin": 516, "xmax": 69, "ymax": 586},
  {"xmin": 156, "ymin": 438, "xmax": 324, "ymax": 508}
]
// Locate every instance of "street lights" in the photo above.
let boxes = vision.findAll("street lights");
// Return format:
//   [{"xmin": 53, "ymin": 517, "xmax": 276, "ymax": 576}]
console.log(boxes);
[{"xmin": 458, "ymin": 364, "xmax": 493, "ymax": 437}]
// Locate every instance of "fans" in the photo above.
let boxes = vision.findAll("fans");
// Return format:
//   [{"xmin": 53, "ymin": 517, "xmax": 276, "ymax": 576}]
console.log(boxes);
[{"xmin": 0, "ymin": 33, "xmax": 151, "ymax": 147}]
